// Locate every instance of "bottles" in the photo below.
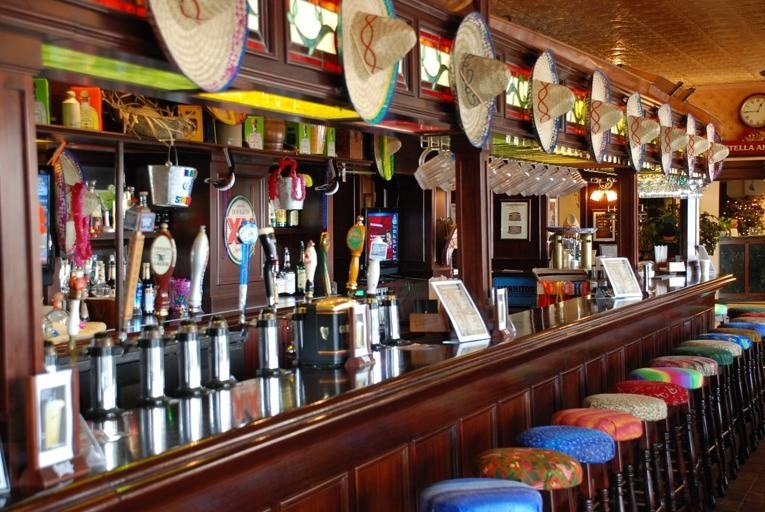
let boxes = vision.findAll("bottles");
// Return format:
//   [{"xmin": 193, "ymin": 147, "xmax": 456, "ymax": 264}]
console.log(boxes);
[
  {"xmin": 142, "ymin": 262, "xmax": 155, "ymax": 316},
  {"xmin": 598, "ymin": 265, "xmax": 608, "ymax": 290},
  {"xmin": 137, "ymin": 402, "xmax": 167, "ymax": 455},
  {"xmin": 127, "ymin": 185, "xmax": 136, "ymax": 207},
  {"xmin": 295, "ymin": 368, "xmax": 304, "ymax": 410},
  {"xmin": 292, "ymin": 308, "xmax": 305, "ymax": 362},
  {"xmin": 205, "ymin": 314, "xmax": 231, "ymax": 386},
  {"xmin": 590, "ymin": 265, "xmax": 598, "ymax": 290},
  {"xmin": 85, "ymin": 177, "xmax": 104, "ymax": 234},
  {"xmin": 177, "ymin": 391, "xmax": 205, "ymax": 442},
  {"xmin": 91, "ymin": 332, "xmax": 118, "ymax": 410},
  {"xmin": 89, "ymin": 411, "xmax": 125, "ymax": 471},
  {"xmin": 86, "ymin": 255, "xmax": 116, "ymax": 298},
  {"xmin": 139, "ymin": 324, "xmax": 166, "ymax": 400},
  {"xmin": 372, "ymin": 346, "xmax": 402, "ymax": 383},
  {"xmin": 385, "ymin": 289, "xmax": 401, "ymax": 341},
  {"xmin": 207, "ymin": 387, "xmax": 233, "ymax": 436},
  {"xmin": 257, "ymin": 375, "xmax": 283, "ymax": 416},
  {"xmin": 138, "ymin": 191, "xmax": 149, "ymax": 207},
  {"xmin": 365, "ymin": 294, "xmax": 379, "ymax": 346},
  {"xmin": 255, "ymin": 307, "xmax": 282, "ymax": 375},
  {"xmin": 135, "ymin": 278, "xmax": 143, "ymax": 317},
  {"xmin": 177, "ymin": 318, "xmax": 200, "ymax": 392}
]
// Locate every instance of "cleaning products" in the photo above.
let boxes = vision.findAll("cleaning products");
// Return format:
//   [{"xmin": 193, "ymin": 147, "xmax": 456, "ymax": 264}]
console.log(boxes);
[{"xmin": 62, "ymin": 89, "xmax": 80, "ymax": 127}]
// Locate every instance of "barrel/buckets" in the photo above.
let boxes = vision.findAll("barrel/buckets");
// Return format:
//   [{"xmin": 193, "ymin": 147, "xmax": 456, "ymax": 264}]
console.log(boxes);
[
  {"xmin": 149, "ymin": 142, "xmax": 195, "ymax": 208},
  {"xmin": 272, "ymin": 155, "xmax": 305, "ymax": 211}
]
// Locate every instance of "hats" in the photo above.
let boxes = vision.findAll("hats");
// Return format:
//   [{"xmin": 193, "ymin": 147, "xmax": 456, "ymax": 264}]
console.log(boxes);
[
  {"xmin": 338, "ymin": 0, "xmax": 419, "ymax": 126},
  {"xmin": 589, "ymin": 68, "xmax": 730, "ymax": 184},
  {"xmin": 452, "ymin": 11, "xmax": 514, "ymax": 149},
  {"xmin": 529, "ymin": 47, "xmax": 577, "ymax": 156},
  {"xmin": 147, "ymin": 2, "xmax": 251, "ymax": 95},
  {"xmin": 373, "ymin": 133, "xmax": 404, "ymax": 182}
]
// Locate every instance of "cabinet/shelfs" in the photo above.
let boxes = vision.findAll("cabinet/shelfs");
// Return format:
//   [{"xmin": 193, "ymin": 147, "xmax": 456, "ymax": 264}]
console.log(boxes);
[{"xmin": 36, "ymin": 139, "xmax": 210, "ymax": 337}]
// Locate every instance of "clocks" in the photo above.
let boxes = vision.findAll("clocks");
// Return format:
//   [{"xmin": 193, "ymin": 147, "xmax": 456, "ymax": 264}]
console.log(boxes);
[{"xmin": 739, "ymin": 92, "xmax": 765, "ymax": 129}]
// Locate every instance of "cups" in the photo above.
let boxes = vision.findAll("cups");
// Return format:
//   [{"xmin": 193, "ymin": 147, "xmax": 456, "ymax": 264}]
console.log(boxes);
[
  {"xmin": 414, "ymin": 143, "xmax": 587, "ymax": 198},
  {"xmin": 700, "ymin": 260, "xmax": 710, "ymax": 274},
  {"xmin": 689, "ymin": 261, "xmax": 701, "ymax": 275},
  {"xmin": 277, "ymin": 175, "xmax": 308, "ymax": 211}
]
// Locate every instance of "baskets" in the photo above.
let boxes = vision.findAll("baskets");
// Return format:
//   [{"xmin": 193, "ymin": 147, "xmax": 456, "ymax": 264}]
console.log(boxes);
[{"xmin": 118, "ymin": 105, "xmax": 181, "ymax": 140}]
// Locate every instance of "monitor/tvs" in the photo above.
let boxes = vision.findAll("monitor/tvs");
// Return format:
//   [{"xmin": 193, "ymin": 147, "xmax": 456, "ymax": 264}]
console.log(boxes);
[{"xmin": 361, "ymin": 207, "xmax": 400, "ymax": 279}]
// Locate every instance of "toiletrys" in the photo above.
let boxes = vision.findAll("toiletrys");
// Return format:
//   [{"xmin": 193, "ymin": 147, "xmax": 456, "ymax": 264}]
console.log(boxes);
[{"xmin": 75, "ymin": 89, "xmax": 99, "ymax": 129}]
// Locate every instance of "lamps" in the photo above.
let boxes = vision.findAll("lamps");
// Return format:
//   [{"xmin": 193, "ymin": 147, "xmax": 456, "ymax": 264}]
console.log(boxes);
[{"xmin": 588, "ymin": 179, "xmax": 620, "ymax": 202}]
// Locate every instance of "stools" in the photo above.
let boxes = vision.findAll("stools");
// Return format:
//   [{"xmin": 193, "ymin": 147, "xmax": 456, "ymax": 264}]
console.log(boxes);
[
  {"xmin": 680, "ymin": 339, "xmax": 748, "ymax": 460},
  {"xmin": 583, "ymin": 392, "xmax": 675, "ymax": 509},
  {"xmin": 630, "ymin": 367, "xmax": 713, "ymax": 512},
  {"xmin": 477, "ymin": 445, "xmax": 587, "ymax": 511},
  {"xmin": 700, "ymin": 304, "xmax": 763, "ymax": 457},
  {"xmin": 553, "ymin": 408, "xmax": 652, "ymax": 510},
  {"xmin": 519, "ymin": 423, "xmax": 619, "ymax": 510},
  {"xmin": 649, "ymin": 355, "xmax": 728, "ymax": 512},
  {"xmin": 675, "ymin": 346, "xmax": 740, "ymax": 479},
  {"xmin": 417, "ymin": 474, "xmax": 544, "ymax": 511},
  {"xmin": 613, "ymin": 378, "xmax": 702, "ymax": 512}
]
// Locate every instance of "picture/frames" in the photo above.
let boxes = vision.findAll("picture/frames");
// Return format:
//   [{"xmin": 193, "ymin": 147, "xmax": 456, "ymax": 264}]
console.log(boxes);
[{"xmin": 499, "ymin": 198, "xmax": 532, "ymax": 242}]
